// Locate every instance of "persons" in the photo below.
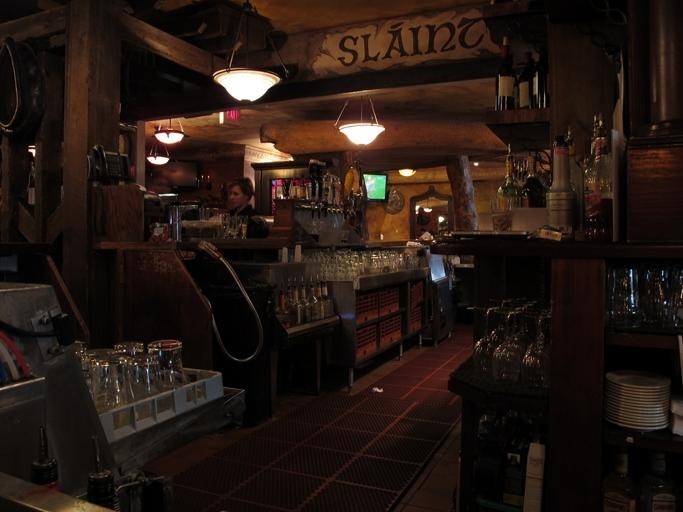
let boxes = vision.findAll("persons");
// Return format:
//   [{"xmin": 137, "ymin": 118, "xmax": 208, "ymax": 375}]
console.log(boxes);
[{"xmin": 227, "ymin": 178, "xmax": 268, "ymax": 238}]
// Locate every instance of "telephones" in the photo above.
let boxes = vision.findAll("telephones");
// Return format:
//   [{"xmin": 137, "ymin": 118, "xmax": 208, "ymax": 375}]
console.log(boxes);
[{"xmin": 92, "ymin": 144, "xmax": 130, "ymax": 178}]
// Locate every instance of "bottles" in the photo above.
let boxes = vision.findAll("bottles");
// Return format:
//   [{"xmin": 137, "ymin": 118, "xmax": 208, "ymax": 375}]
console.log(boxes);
[
  {"xmin": 545, "ymin": 141, "xmax": 576, "ymax": 242},
  {"xmin": 494, "ymin": 28, "xmax": 549, "ymax": 110},
  {"xmin": 276, "ymin": 274, "xmax": 333, "ymax": 328},
  {"xmin": 496, "ymin": 152, "xmax": 546, "ymax": 210},
  {"xmin": 468, "ymin": 406, "xmax": 683, "ymax": 508},
  {"xmin": 584, "ymin": 113, "xmax": 613, "ymax": 243}
]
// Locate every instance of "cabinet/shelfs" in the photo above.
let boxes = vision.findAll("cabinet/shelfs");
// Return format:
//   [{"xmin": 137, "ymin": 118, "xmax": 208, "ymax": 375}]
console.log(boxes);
[
  {"xmin": 438, "ymin": 135, "xmax": 683, "ymax": 510},
  {"xmin": 103, "ymin": 186, "xmax": 457, "ymax": 413}
]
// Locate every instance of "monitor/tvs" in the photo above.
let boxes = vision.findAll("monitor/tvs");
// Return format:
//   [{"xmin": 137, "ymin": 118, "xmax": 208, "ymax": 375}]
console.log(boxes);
[
  {"xmin": 160, "ymin": 159, "xmax": 204, "ymax": 191},
  {"xmin": 358, "ymin": 173, "xmax": 388, "ymax": 203}
]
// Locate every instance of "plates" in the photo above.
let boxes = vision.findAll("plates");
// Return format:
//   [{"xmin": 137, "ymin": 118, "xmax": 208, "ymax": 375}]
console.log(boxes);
[{"xmin": 605, "ymin": 371, "xmax": 672, "ymax": 434}]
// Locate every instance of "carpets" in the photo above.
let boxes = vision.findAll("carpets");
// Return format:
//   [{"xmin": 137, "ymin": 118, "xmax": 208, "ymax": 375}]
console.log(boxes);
[{"xmin": 156, "ymin": 317, "xmax": 474, "ymax": 509}]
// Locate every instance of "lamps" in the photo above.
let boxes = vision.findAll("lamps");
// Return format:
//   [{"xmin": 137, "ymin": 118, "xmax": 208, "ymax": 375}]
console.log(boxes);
[
  {"xmin": 335, "ymin": 97, "xmax": 388, "ymax": 152},
  {"xmin": 154, "ymin": 120, "xmax": 186, "ymax": 145},
  {"xmin": 211, "ymin": 0, "xmax": 287, "ymax": 107},
  {"xmin": 147, "ymin": 135, "xmax": 171, "ymax": 167}
]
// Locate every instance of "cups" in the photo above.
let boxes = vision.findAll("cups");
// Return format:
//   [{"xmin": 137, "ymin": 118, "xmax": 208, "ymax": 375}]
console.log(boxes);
[
  {"xmin": 71, "ymin": 341, "xmax": 189, "ymax": 416},
  {"xmin": 607, "ymin": 261, "xmax": 683, "ymax": 333},
  {"xmin": 307, "ymin": 248, "xmax": 413, "ymax": 283},
  {"xmin": 225, "ymin": 214, "xmax": 248, "ymax": 241}
]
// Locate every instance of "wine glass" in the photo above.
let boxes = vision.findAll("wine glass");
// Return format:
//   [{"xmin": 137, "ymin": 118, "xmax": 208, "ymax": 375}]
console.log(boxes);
[{"xmin": 472, "ymin": 297, "xmax": 551, "ymax": 388}]
